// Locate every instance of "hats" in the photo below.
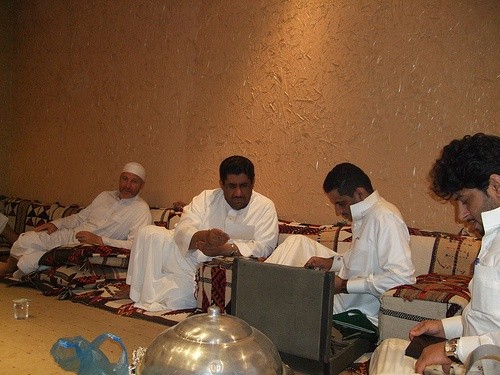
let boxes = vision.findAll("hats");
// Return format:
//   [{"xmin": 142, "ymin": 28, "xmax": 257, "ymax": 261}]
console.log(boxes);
[{"xmin": 120, "ymin": 161, "xmax": 145, "ymax": 181}]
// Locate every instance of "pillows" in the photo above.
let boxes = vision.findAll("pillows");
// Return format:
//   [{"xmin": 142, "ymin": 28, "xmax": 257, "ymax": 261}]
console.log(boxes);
[{"xmin": 21, "ymin": 242, "xmax": 130, "ymax": 306}]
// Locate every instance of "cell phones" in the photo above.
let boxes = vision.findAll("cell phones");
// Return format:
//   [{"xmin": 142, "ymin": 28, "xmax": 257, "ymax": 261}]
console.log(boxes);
[{"xmin": 306, "ymin": 263, "xmax": 314, "ymax": 270}]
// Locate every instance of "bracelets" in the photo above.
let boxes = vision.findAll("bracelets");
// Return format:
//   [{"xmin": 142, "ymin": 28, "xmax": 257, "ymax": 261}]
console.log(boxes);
[
  {"xmin": 231, "ymin": 243, "xmax": 238, "ymax": 257},
  {"xmin": 341, "ymin": 279, "xmax": 348, "ymax": 294}
]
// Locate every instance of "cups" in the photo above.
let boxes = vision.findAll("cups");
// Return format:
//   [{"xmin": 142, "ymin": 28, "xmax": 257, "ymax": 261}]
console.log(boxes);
[{"xmin": 14, "ymin": 298, "xmax": 29, "ymax": 320}]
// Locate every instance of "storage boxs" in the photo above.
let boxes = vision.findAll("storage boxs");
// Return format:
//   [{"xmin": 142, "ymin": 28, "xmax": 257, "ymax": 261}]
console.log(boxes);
[{"xmin": 231, "ymin": 257, "xmax": 370, "ymax": 375}]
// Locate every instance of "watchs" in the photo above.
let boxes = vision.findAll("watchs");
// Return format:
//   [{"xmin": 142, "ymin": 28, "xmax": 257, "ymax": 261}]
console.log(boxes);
[{"xmin": 445, "ymin": 337, "xmax": 464, "ymax": 365}]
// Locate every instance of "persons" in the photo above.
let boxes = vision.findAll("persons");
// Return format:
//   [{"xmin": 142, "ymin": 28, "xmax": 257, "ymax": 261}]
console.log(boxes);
[
  {"xmin": 0, "ymin": 161, "xmax": 153, "ymax": 280},
  {"xmin": 0, "ymin": 213, "xmax": 20, "ymax": 245},
  {"xmin": 112, "ymin": 155, "xmax": 279, "ymax": 312},
  {"xmin": 368, "ymin": 133, "xmax": 500, "ymax": 375},
  {"xmin": 263, "ymin": 163, "xmax": 417, "ymax": 327}
]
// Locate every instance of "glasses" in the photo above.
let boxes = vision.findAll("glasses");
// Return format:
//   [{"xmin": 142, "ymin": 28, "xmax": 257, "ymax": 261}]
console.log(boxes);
[{"xmin": 120, "ymin": 175, "xmax": 140, "ymax": 185}]
